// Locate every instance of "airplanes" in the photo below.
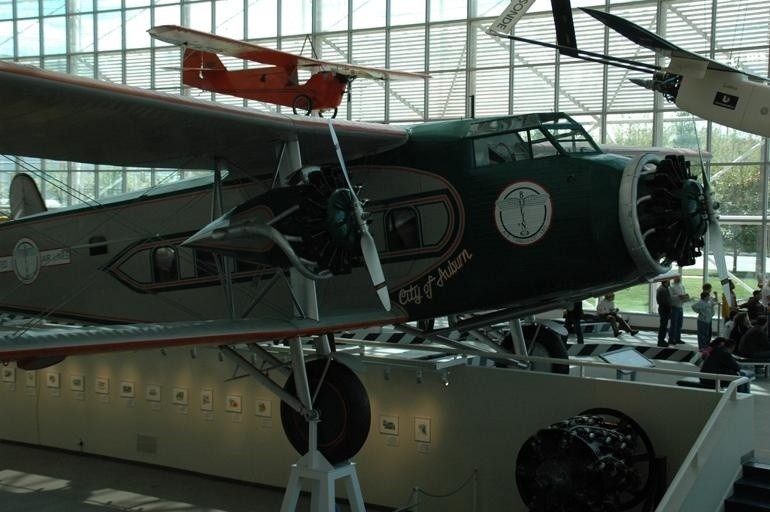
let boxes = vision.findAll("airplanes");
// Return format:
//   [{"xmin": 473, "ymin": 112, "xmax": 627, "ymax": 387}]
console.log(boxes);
[
  {"xmin": 0, "ymin": 0, "xmax": 721, "ymax": 466},
  {"xmin": 575, "ymin": 5, "xmax": 770, "ymax": 142}
]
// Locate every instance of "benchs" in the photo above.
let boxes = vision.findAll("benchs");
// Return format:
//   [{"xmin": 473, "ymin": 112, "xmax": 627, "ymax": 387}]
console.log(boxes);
[{"xmin": 552, "ymin": 314, "xmax": 629, "ymax": 333}]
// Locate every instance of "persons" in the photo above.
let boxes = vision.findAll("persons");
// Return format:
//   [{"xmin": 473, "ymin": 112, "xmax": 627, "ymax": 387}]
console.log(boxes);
[
  {"xmin": 667, "ymin": 274, "xmax": 691, "ymax": 345},
  {"xmin": 596, "ymin": 291, "xmax": 639, "ymax": 338},
  {"xmin": 700, "ymin": 336, "xmax": 726, "ymax": 363},
  {"xmin": 560, "ymin": 300, "xmax": 584, "ymax": 345},
  {"xmin": 702, "ymin": 282, "xmax": 719, "ymax": 344},
  {"xmin": 691, "ymin": 291, "xmax": 717, "ymax": 352},
  {"xmin": 655, "ymin": 279, "xmax": 675, "ymax": 347},
  {"xmin": 721, "ymin": 278, "xmax": 770, "ymax": 375},
  {"xmin": 698, "ymin": 338, "xmax": 749, "ymax": 393}
]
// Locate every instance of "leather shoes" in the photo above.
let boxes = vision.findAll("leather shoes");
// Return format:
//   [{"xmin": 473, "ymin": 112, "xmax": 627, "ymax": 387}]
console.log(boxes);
[
  {"xmin": 658, "ymin": 340, "xmax": 685, "ymax": 347},
  {"xmin": 615, "ymin": 332, "xmax": 622, "ymax": 337},
  {"xmin": 631, "ymin": 330, "xmax": 638, "ymax": 336}
]
